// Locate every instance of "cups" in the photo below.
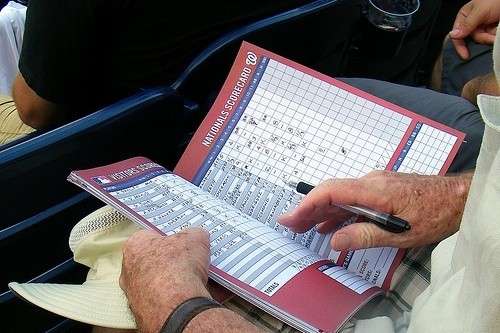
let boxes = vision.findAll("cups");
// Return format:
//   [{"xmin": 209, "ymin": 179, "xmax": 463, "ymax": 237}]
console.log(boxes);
[{"xmin": 367, "ymin": 0, "xmax": 420, "ymax": 32}]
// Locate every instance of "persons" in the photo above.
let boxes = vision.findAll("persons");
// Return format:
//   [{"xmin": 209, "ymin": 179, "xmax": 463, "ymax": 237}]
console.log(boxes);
[
  {"xmin": 118, "ymin": 23, "xmax": 500, "ymax": 333},
  {"xmin": 331, "ymin": 0, "xmax": 500, "ymax": 174},
  {"xmin": 11, "ymin": 0, "xmax": 316, "ymax": 144}
]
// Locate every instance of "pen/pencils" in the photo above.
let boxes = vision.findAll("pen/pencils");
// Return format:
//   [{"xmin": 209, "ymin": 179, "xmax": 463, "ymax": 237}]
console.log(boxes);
[{"xmin": 283, "ymin": 178, "xmax": 412, "ymax": 234}]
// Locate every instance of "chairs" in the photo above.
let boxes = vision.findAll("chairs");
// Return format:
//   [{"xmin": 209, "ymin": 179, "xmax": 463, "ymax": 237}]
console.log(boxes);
[
  {"xmin": 172, "ymin": 0, "xmax": 443, "ymax": 138},
  {"xmin": 0, "ymin": 87, "xmax": 179, "ymax": 333}
]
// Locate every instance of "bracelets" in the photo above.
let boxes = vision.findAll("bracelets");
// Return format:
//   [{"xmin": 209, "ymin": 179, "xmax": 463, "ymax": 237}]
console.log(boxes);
[{"xmin": 155, "ymin": 297, "xmax": 229, "ymax": 333}]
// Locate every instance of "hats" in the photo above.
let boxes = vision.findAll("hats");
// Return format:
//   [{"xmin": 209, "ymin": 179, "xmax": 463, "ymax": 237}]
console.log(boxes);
[{"xmin": 8, "ymin": 205, "xmax": 141, "ymax": 329}]
